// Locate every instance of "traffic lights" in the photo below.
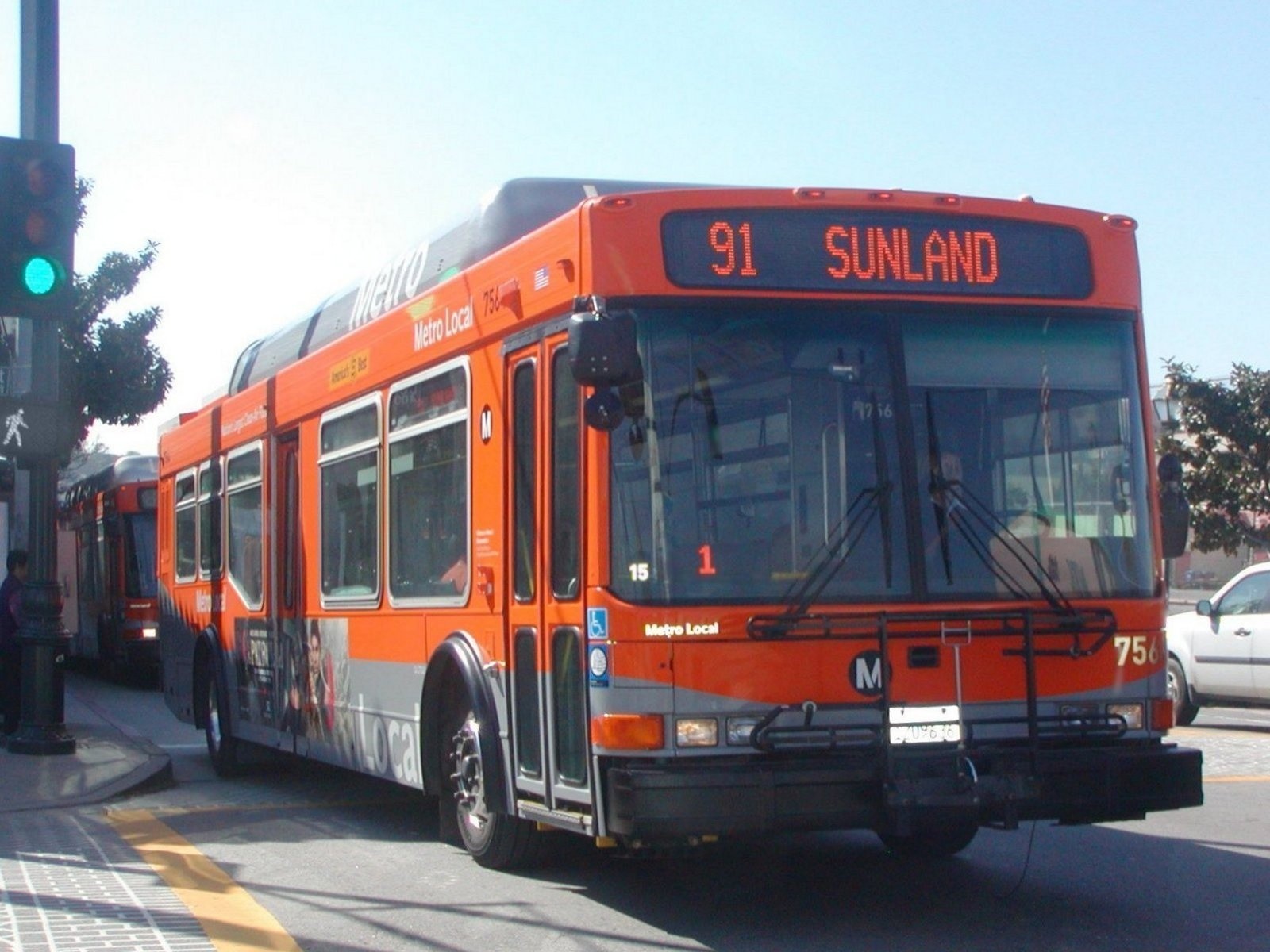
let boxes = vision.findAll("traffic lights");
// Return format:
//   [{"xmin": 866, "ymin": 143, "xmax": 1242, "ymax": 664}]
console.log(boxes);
[
  {"xmin": 0, "ymin": 399, "xmax": 44, "ymax": 458},
  {"xmin": 0, "ymin": 136, "xmax": 76, "ymax": 321}
]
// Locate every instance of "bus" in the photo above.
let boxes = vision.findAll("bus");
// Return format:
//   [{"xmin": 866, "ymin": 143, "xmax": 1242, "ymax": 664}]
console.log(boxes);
[
  {"xmin": 157, "ymin": 178, "xmax": 1203, "ymax": 870},
  {"xmin": 63, "ymin": 454, "xmax": 160, "ymax": 689}
]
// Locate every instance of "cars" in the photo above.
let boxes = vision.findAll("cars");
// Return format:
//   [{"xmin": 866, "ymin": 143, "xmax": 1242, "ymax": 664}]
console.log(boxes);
[{"xmin": 1164, "ymin": 560, "xmax": 1270, "ymax": 727}]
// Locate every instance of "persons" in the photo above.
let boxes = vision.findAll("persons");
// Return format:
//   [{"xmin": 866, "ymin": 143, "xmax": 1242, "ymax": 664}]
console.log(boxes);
[
  {"xmin": 920, "ymin": 445, "xmax": 979, "ymax": 577},
  {"xmin": 0, "ymin": 547, "xmax": 27, "ymax": 736},
  {"xmin": 440, "ymin": 529, "xmax": 467, "ymax": 594}
]
